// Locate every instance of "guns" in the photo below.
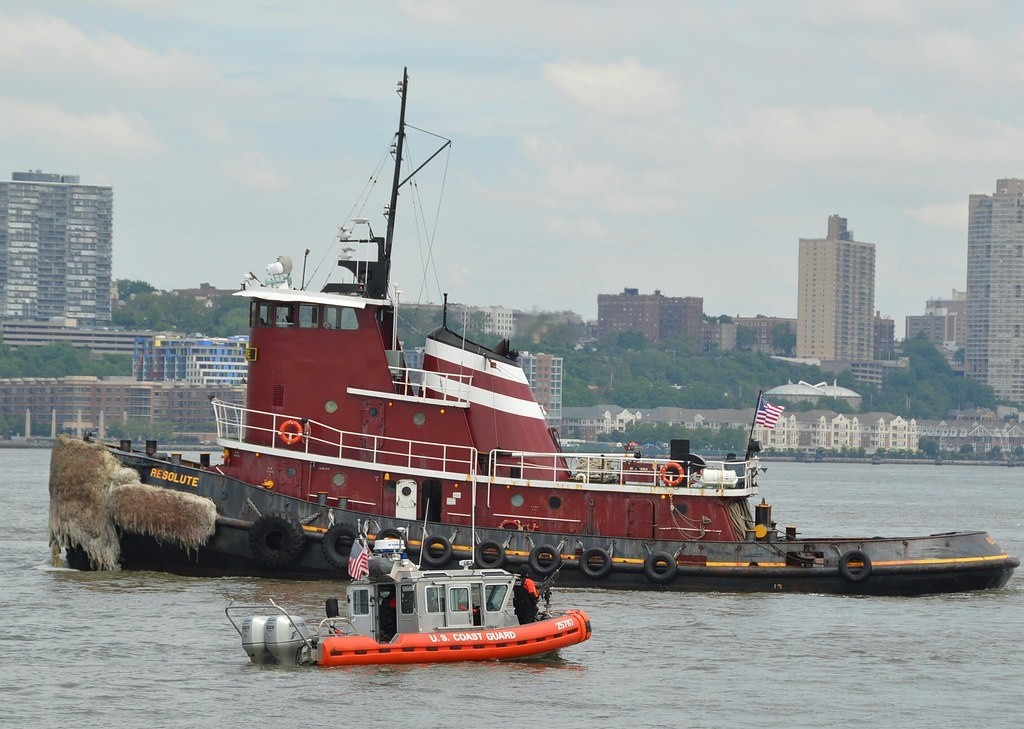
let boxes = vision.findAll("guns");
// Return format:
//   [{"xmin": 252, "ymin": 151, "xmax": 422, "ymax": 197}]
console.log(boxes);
[{"xmin": 538, "ymin": 562, "xmax": 565, "ymax": 599}]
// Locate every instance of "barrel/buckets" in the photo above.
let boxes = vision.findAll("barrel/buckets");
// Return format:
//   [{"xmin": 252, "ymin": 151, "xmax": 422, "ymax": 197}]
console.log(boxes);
[
  {"xmin": 146, "ymin": 439, "xmax": 157, "ymax": 458},
  {"xmin": 120, "ymin": 439, "xmax": 131, "ymax": 453},
  {"xmin": 746, "ymin": 529, "xmax": 757, "ymax": 541},
  {"xmin": 765, "ymin": 530, "xmax": 778, "ymax": 542},
  {"xmin": 171, "ymin": 453, "xmax": 181, "ymax": 467},
  {"xmin": 785, "ymin": 526, "xmax": 796, "ymax": 542},
  {"xmin": 317, "ymin": 491, "xmax": 328, "ymax": 506},
  {"xmin": 337, "ymin": 495, "xmax": 349, "ymax": 510},
  {"xmin": 200, "ymin": 453, "xmax": 210, "ymax": 472}
]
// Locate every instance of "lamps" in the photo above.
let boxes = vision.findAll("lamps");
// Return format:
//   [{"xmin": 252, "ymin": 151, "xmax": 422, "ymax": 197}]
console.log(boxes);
[{"xmin": 458, "ymin": 560, "xmax": 475, "ymax": 569}]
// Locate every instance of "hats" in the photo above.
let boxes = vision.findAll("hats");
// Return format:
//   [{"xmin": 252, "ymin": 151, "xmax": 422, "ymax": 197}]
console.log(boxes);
[{"xmin": 520, "ymin": 564, "xmax": 530, "ymax": 572}]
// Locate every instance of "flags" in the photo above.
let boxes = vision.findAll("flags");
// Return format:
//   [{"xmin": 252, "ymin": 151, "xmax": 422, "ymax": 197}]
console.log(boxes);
[
  {"xmin": 753, "ymin": 394, "xmax": 787, "ymax": 429},
  {"xmin": 347, "ymin": 538, "xmax": 373, "ymax": 581}
]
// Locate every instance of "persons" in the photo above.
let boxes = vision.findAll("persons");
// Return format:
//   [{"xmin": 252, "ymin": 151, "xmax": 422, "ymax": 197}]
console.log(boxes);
[
  {"xmin": 381, "ymin": 587, "xmax": 397, "ymax": 635},
  {"xmin": 512, "ymin": 564, "xmax": 542, "ymax": 625},
  {"xmin": 632, "ymin": 450, "xmax": 641, "ymax": 470}
]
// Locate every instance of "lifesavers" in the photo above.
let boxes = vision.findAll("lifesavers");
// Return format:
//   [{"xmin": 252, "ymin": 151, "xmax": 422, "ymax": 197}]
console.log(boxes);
[
  {"xmin": 644, "ymin": 551, "xmax": 676, "ymax": 582},
  {"xmin": 321, "ymin": 523, "xmax": 366, "ymax": 569},
  {"xmin": 528, "ymin": 544, "xmax": 561, "ymax": 574},
  {"xmin": 661, "ymin": 462, "xmax": 685, "ymax": 486},
  {"xmin": 839, "ymin": 550, "xmax": 871, "ymax": 581},
  {"xmin": 580, "ymin": 549, "xmax": 610, "ymax": 577},
  {"xmin": 421, "ymin": 534, "xmax": 453, "ymax": 562},
  {"xmin": 476, "ymin": 538, "xmax": 505, "ymax": 569},
  {"xmin": 279, "ymin": 421, "xmax": 303, "ymax": 445},
  {"xmin": 247, "ymin": 511, "xmax": 304, "ymax": 565},
  {"xmin": 374, "ymin": 529, "xmax": 405, "ymax": 558}
]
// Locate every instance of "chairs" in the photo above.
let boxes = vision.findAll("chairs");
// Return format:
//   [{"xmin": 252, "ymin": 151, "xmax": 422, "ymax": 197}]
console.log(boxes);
[{"xmin": 379, "ymin": 605, "xmax": 392, "ymax": 639}]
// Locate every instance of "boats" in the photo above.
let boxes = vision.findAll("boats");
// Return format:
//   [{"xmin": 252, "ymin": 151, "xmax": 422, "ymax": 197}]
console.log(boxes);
[
  {"xmin": 35, "ymin": 63, "xmax": 1020, "ymax": 599},
  {"xmin": 223, "ymin": 564, "xmax": 595, "ymax": 668}
]
